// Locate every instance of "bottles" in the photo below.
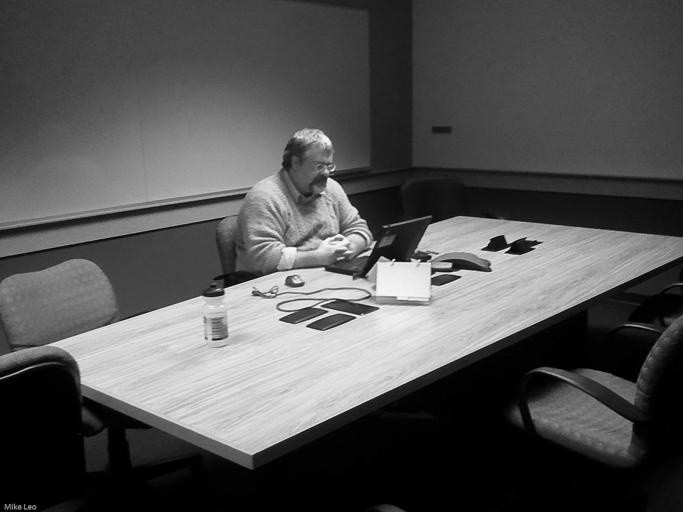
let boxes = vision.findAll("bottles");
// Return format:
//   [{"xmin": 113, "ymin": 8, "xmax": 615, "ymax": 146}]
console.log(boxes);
[{"xmin": 202, "ymin": 282, "xmax": 230, "ymax": 349}]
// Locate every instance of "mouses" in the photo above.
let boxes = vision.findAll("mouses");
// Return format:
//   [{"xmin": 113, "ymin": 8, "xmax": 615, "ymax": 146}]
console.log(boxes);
[{"xmin": 286, "ymin": 275, "xmax": 304, "ymax": 286}]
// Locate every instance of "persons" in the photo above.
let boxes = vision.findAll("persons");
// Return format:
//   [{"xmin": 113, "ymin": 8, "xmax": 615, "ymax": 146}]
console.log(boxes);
[{"xmin": 233, "ymin": 127, "xmax": 374, "ymax": 283}]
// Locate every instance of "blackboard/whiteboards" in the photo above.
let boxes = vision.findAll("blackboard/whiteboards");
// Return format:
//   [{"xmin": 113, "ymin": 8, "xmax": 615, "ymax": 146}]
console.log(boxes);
[{"xmin": 1, "ymin": 0, "xmax": 375, "ymax": 235}]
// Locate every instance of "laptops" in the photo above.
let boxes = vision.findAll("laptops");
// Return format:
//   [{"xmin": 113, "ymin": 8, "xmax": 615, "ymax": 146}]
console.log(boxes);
[{"xmin": 326, "ymin": 215, "xmax": 433, "ymax": 278}]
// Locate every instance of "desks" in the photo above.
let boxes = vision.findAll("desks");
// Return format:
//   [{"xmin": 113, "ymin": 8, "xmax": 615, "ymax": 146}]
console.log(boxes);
[{"xmin": 40, "ymin": 212, "xmax": 682, "ymax": 511}]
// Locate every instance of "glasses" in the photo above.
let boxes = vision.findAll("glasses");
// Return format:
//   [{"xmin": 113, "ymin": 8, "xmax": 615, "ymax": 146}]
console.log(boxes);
[{"xmin": 307, "ymin": 157, "xmax": 337, "ymax": 172}]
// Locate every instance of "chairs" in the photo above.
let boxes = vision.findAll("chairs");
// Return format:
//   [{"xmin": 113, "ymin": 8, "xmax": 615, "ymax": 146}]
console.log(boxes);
[
  {"xmin": 209, "ymin": 214, "xmax": 254, "ymax": 285},
  {"xmin": 0, "ymin": 345, "xmax": 88, "ymax": 511},
  {"xmin": 400, "ymin": 171, "xmax": 505, "ymax": 222},
  {"xmin": 503, "ymin": 313, "xmax": 681, "ymax": 511},
  {"xmin": 0, "ymin": 258, "xmax": 153, "ymax": 484}
]
